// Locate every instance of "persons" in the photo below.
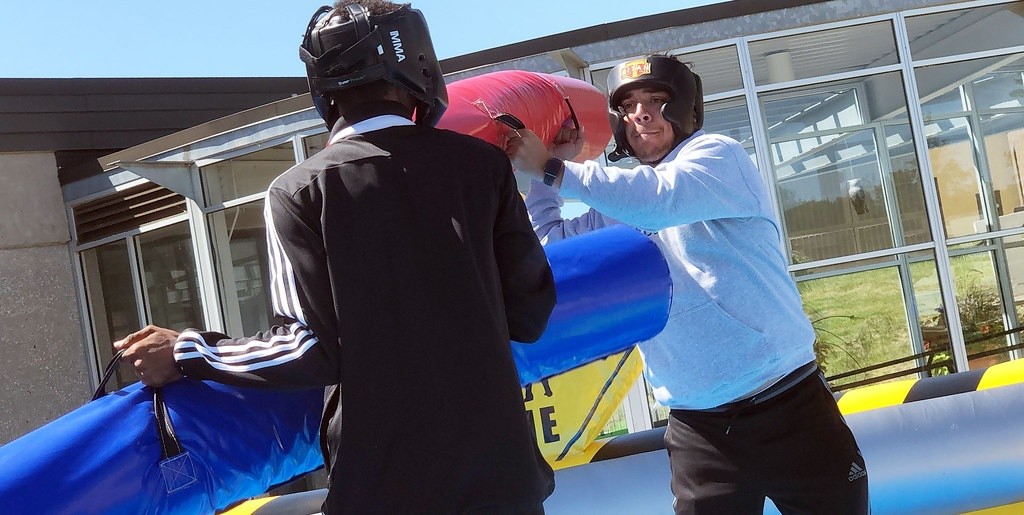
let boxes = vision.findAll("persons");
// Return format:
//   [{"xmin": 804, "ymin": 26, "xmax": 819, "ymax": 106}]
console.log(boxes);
[
  {"xmin": 112, "ymin": 0, "xmax": 558, "ymax": 515},
  {"xmin": 504, "ymin": 56, "xmax": 872, "ymax": 515}
]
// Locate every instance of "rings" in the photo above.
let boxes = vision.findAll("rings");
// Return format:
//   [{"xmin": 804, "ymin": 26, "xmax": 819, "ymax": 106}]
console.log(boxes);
[{"xmin": 138, "ymin": 372, "xmax": 145, "ymax": 377}]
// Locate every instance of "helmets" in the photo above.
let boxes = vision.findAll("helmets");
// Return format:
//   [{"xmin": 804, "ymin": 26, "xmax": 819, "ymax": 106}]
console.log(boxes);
[
  {"xmin": 606, "ymin": 54, "xmax": 704, "ymax": 163},
  {"xmin": 298, "ymin": 3, "xmax": 449, "ymax": 132}
]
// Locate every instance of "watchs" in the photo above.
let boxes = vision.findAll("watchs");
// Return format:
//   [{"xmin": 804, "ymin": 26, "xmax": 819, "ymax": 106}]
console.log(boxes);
[{"xmin": 543, "ymin": 158, "xmax": 563, "ymax": 185}]
